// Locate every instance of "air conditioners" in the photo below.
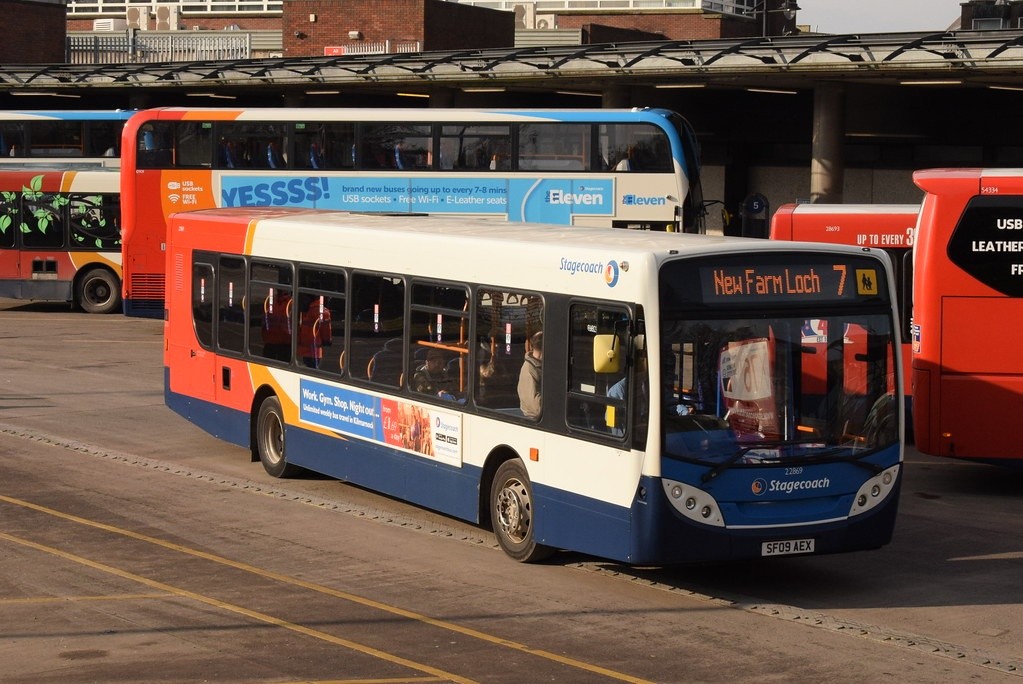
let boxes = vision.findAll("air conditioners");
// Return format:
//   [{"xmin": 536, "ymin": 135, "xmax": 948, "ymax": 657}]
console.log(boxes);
[
  {"xmin": 126, "ymin": 7, "xmax": 150, "ymax": 31},
  {"xmin": 512, "ymin": 2, "xmax": 558, "ymax": 30},
  {"xmin": 156, "ymin": 6, "xmax": 181, "ymax": 31}
]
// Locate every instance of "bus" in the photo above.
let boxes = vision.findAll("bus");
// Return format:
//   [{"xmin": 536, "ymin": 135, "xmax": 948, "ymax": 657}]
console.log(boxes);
[
  {"xmin": 0, "ymin": 108, "xmax": 156, "ymax": 315},
  {"xmin": 122, "ymin": 106, "xmax": 733, "ymax": 319},
  {"xmin": 767, "ymin": 201, "xmax": 923, "ymax": 443},
  {"xmin": 909, "ymin": 167, "xmax": 1023, "ymax": 466},
  {"xmin": 161, "ymin": 205, "xmax": 907, "ymax": 568}
]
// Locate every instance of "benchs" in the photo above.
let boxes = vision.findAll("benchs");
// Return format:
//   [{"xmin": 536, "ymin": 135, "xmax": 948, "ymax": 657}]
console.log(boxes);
[
  {"xmin": 12, "ymin": 145, "xmax": 83, "ymax": 156},
  {"xmin": 494, "ymin": 154, "xmax": 585, "ymax": 170}
]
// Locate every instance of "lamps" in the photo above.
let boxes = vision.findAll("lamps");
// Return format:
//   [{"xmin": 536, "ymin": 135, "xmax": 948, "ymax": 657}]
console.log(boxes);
[{"xmin": 349, "ymin": 31, "xmax": 359, "ymax": 40}]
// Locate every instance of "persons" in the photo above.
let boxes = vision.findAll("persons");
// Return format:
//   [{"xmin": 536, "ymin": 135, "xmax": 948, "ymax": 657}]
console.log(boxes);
[
  {"xmin": 608, "ymin": 350, "xmax": 694, "ymax": 437},
  {"xmin": 286, "ymin": 293, "xmax": 331, "ymax": 368},
  {"xmin": 363, "ymin": 288, "xmax": 575, "ymax": 421}
]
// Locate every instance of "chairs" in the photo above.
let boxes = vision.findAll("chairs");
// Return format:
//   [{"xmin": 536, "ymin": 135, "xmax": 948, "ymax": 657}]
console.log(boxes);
[
  {"xmin": 218, "ymin": 135, "xmax": 482, "ymax": 169},
  {"xmin": 144, "ymin": 131, "xmax": 170, "ymax": 162},
  {"xmin": 242, "ymin": 295, "xmax": 460, "ymax": 403}
]
